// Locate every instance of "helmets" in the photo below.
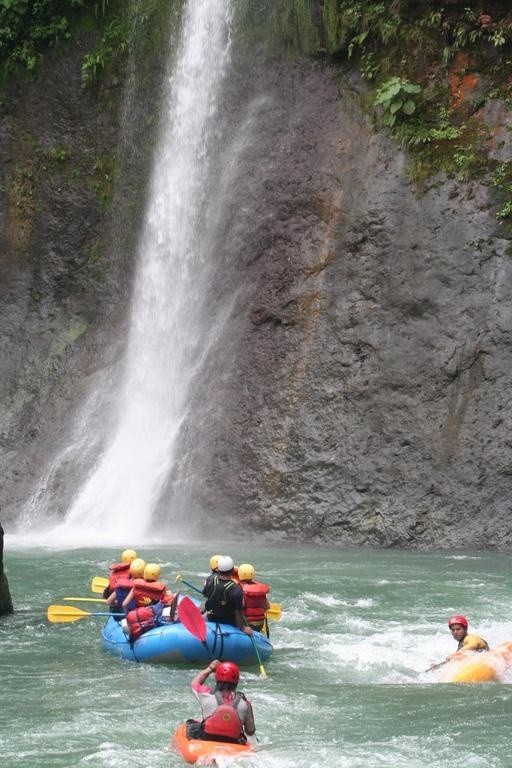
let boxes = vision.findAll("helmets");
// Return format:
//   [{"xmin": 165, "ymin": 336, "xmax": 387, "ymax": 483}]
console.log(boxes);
[
  {"xmin": 449, "ymin": 617, "xmax": 468, "ymax": 630},
  {"xmin": 122, "ymin": 550, "xmax": 160, "ymax": 581},
  {"xmin": 215, "ymin": 662, "xmax": 239, "ymax": 686},
  {"xmin": 209, "ymin": 554, "xmax": 254, "ymax": 581}
]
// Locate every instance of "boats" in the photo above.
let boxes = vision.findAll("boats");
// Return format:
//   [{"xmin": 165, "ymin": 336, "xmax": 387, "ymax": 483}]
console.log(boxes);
[
  {"xmin": 449, "ymin": 639, "xmax": 512, "ymax": 685},
  {"xmin": 100, "ymin": 590, "xmax": 280, "ymax": 664},
  {"xmin": 170, "ymin": 715, "xmax": 255, "ymax": 766}
]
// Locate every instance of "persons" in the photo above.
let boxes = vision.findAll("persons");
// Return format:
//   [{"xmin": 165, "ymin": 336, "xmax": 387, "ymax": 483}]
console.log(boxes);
[
  {"xmin": 186, "ymin": 659, "xmax": 256, "ymax": 744},
  {"xmin": 430, "ymin": 616, "xmax": 490, "ymax": 672},
  {"xmin": 104, "ymin": 550, "xmax": 271, "ymax": 637}
]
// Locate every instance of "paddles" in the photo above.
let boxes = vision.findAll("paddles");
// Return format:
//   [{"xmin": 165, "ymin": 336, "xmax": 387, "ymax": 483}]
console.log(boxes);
[
  {"xmin": 92, "ymin": 576, "xmax": 110, "ymax": 593},
  {"xmin": 178, "ymin": 595, "xmax": 214, "ymax": 660},
  {"xmin": 62, "ymin": 596, "xmax": 108, "ymax": 602},
  {"xmin": 265, "ymin": 603, "xmax": 282, "ymax": 619},
  {"xmin": 47, "ymin": 605, "xmax": 126, "ymax": 623}
]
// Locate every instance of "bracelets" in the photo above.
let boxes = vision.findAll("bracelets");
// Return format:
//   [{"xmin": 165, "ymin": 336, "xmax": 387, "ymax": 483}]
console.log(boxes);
[{"xmin": 207, "ymin": 664, "xmax": 214, "ymax": 671}]
